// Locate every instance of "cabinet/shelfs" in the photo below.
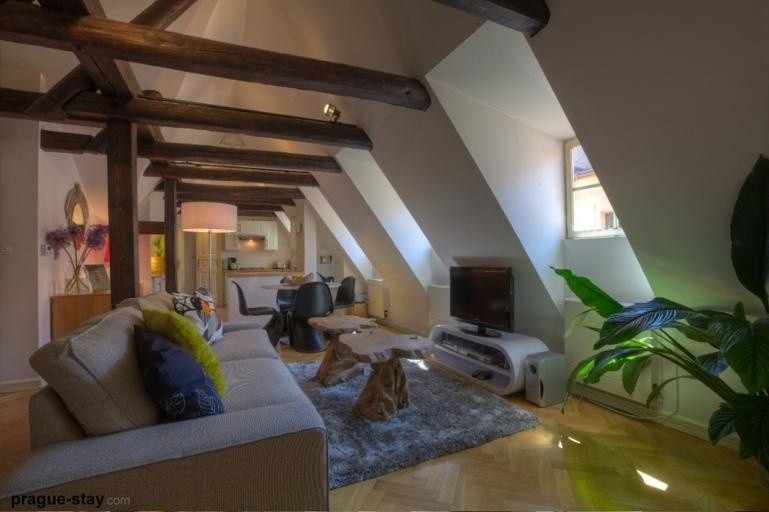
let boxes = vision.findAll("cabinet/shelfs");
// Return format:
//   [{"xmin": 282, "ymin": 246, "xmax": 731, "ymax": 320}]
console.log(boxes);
[{"xmin": 428, "ymin": 323, "xmax": 552, "ymax": 398}]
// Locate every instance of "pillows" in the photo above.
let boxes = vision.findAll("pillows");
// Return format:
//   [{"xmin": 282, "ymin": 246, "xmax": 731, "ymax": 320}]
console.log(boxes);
[{"xmin": 134, "ymin": 285, "xmax": 225, "ymax": 423}]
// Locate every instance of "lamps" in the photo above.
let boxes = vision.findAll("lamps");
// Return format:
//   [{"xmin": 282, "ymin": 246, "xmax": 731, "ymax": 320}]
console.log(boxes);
[
  {"xmin": 180, "ymin": 201, "xmax": 239, "ymax": 296},
  {"xmin": 322, "ymin": 102, "xmax": 342, "ymax": 123}
]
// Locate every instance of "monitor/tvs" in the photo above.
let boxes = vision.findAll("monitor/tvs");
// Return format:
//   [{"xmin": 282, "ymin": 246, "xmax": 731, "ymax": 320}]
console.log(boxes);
[{"xmin": 449, "ymin": 266, "xmax": 514, "ymax": 336}]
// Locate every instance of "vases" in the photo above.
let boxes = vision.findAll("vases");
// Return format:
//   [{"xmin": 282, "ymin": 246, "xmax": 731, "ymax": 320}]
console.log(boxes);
[{"xmin": 65, "ymin": 264, "xmax": 89, "ymax": 296}]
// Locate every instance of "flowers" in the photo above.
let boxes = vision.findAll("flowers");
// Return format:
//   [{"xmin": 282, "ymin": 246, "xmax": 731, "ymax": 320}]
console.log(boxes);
[{"xmin": 46, "ymin": 222, "xmax": 112, "ymax": 293}]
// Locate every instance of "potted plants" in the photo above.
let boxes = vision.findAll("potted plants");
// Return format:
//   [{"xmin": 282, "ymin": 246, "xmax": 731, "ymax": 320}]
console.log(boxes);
[{"xmin": 149, "ymin": 234, "xmax": 167, "ymax": 275}]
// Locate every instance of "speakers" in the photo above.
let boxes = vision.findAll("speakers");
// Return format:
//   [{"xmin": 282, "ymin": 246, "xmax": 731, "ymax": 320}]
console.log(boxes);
[{"xmin": 525, "ymin": 351, "xmax": 568, "ymax": 408}]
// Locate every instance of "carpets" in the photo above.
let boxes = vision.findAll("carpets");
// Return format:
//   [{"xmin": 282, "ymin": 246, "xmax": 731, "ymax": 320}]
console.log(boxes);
[{"xmin": 285, "ymin": 356, "xmax": 542, "ymax": 490}]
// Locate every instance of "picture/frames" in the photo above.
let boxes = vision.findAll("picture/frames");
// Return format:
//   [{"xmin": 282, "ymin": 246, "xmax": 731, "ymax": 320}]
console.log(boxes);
[{"xmin": 83, "ymin": 263, "xmax": 111, "ymax": 292}]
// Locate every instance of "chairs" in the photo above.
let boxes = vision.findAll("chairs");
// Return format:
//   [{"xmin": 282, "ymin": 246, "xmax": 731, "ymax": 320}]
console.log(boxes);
[{"xmin": 231, "ymin": 269, "xmax": 356, "ymax": 353}]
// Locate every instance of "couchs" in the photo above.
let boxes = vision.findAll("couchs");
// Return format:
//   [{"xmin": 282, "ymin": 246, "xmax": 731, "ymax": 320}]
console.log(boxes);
[{"xmin": 1, "ymin": 291, "xmax": 330, "ymax": 512}]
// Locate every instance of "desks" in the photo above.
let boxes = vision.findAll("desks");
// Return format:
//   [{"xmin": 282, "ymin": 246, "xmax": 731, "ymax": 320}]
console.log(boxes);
[{"xmin": 308, "ymin": 312, "xmax": 435, "ymax": 421}]
